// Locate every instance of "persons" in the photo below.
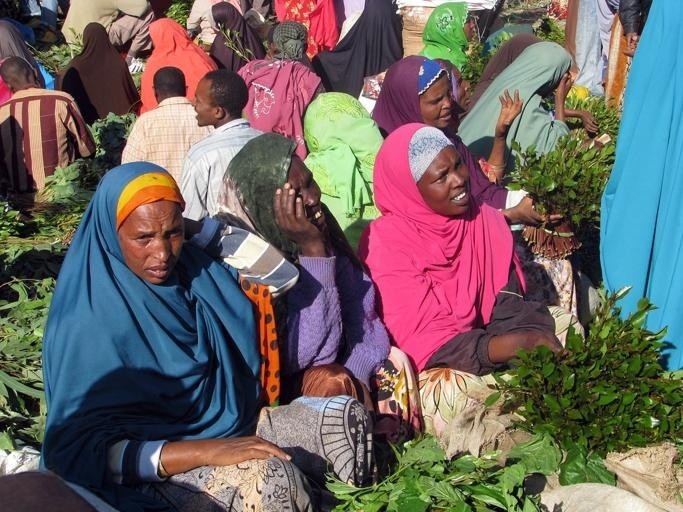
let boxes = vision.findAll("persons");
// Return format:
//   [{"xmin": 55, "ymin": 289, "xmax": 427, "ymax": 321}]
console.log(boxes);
[{"xmin": 2, "ymin": 1, "xmax": 683, "ymax": 512}]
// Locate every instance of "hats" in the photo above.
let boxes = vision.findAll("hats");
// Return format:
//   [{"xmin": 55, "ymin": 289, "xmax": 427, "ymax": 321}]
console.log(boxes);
[{"xmin": 272, "ymin": 22, "xmax": 308, "ymax": 59}]
